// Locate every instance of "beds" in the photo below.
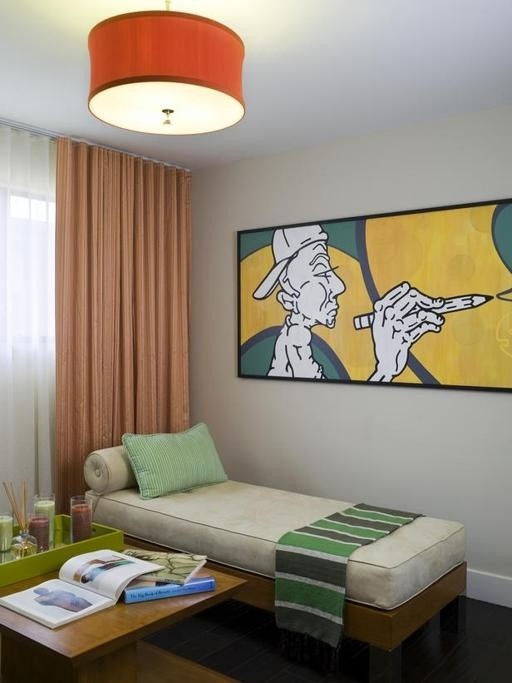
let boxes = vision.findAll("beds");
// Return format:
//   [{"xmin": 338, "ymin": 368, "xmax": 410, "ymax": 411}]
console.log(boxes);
[{"xmin": 81, "ymin": 446, "xmax": 467, "ymax": 683}]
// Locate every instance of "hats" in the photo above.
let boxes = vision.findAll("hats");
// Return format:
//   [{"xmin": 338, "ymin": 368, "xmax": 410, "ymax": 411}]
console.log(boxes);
[{"xmin": 251, "ymin": 223, "xmax": 327, "ymax": 302}]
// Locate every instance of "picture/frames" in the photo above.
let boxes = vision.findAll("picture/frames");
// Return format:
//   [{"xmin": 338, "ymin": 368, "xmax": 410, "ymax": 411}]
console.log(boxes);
[{"xmin": 236, "ymin": 198, "xmax": 512, "ymax": 392}]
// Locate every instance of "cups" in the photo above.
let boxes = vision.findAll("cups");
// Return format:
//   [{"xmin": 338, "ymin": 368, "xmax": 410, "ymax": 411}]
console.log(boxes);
[{"xmin": 0, "ymin": 492, "xmax": 93, "ymax": 561}]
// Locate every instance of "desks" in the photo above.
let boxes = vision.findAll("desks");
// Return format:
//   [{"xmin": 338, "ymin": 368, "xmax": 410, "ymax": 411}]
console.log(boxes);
[{"xmin": 0, "ymin": 543, "xmax": 247, "ymax": 683}]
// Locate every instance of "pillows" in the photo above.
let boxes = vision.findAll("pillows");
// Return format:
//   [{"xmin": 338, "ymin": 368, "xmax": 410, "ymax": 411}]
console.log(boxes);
[{"xmin": 122, "ymin": 422, "xmax": 228, "ymax": 500}]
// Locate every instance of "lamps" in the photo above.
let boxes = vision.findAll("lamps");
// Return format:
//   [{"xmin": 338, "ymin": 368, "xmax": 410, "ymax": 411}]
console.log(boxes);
[{"xmin": 86, "ymin": 1, "xmax": 246, "ymax": 135}]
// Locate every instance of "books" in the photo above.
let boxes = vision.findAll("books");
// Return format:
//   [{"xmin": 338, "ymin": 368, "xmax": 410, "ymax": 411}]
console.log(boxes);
[
  {"xmin": 116, "ymin": 549, "xmax": 210, "ymax": 588},
  {"xmin": 123, "ymin": 576, "xmax": 216, "ymax": 606},
  {"xmin": 2, "ymin": 547, "xmax": 166, "ymax": 633}
]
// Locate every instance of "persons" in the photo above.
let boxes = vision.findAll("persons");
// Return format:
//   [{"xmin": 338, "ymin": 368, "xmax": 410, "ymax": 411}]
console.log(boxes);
[
  {"xmin": 80, "ymin": 559, "xmax": 133, "ymax": 583},
  {"xmin": 31, "ymin": 587, "xmax": 94, "ymax": 612}
]
